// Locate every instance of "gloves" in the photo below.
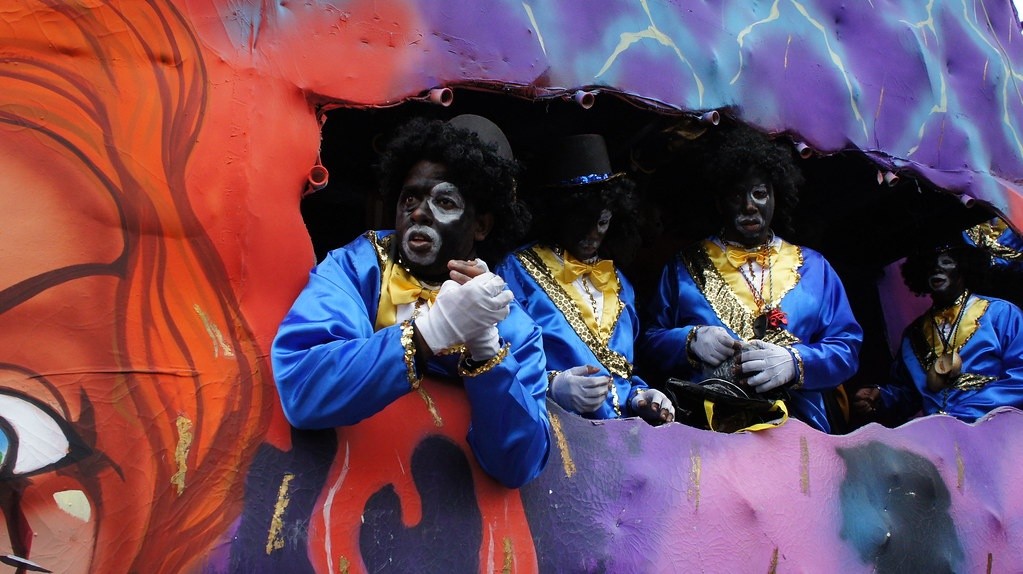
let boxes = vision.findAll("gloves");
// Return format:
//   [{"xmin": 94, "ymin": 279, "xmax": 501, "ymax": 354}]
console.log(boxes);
[{"xmin": 414, "ymin": 271, "xmax": 515, "ymax": 355}]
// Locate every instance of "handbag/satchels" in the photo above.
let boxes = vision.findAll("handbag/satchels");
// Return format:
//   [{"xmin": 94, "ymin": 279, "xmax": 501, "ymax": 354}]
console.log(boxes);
[{"xmin": 665, "ymin": 377, "xmax": 788, "ymax": 434}]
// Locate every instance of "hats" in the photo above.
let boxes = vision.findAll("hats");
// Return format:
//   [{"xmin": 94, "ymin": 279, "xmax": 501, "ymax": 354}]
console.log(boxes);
[
  {"xmin": 540, "ymin": 133, "xmax": 627, "ymax": 189},
  {"xmin": 445, "ymin": 113, "xmax": 514, "ymax": 169}
]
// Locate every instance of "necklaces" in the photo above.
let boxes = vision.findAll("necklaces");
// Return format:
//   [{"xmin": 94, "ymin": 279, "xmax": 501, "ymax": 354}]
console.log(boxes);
[
  {"xmin": 928, "ymin": 291, "xmax": 972, "ymax": 390},
  {"xmin": 719, "ymin": 234, "xmax": 773, "ymax": 314}
]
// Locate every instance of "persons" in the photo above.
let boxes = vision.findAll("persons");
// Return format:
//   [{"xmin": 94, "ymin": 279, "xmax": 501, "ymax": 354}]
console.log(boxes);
[
  {"xmin": 271, "ymin": 111, "xmax": 551, "ymax": 491},
  {"xmin": 854, "ymin": 199, "xmax": 1023, "ymax": 427},
  {"xmin": 493, "ymin": 133, "xmax": 677, "ymax": 422},
  {"xmin": 640, "ymin": 130, "xmax": 862, "ymax": 431}
]
